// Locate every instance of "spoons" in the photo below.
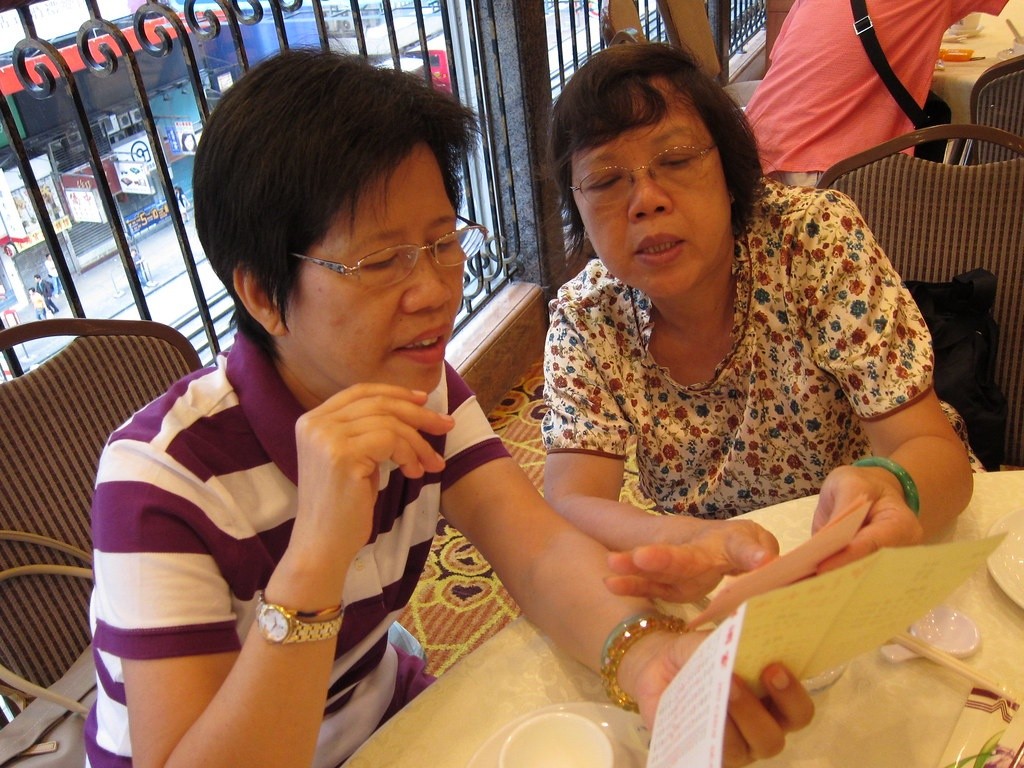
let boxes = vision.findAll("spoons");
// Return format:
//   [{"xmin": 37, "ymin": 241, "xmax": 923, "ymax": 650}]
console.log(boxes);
[{"xmin": 881, "ymin": 604, "xmax": 982, "ymax": 664}]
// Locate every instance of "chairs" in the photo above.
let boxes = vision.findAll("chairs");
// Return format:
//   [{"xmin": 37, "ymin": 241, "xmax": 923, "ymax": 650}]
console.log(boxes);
[
  {"xmin": 817, "ymin": 123, "xmax": 1024, "ymax": 486},
  {"xmin": 600, "ymin": 0, "xmax": 647, "ymax": 51},
  {"xmin": 0, "ymin": 318, "xmax": 205, "ymax": 717},
  {"xmin": 956, "ymin": 54, "xmax": 1024, "ymax": 163},
  {"xmin": 658, "ymin": 0, "xmax": 765, "ymax": 112}
]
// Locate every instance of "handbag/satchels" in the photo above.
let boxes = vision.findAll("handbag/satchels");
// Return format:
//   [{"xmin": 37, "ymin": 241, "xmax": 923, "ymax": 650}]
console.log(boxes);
[{"xmin": 913, "ymin": 91, "xmax": 951, "ymax": 163}]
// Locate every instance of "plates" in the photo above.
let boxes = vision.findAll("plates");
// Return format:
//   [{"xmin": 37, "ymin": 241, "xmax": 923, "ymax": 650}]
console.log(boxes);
[
  {"xmin": 987, "ymin": 506, "xmax": 1024, "ymax": 611},
  {"xmin": 464, "ymin": 701, "xmax": 651, "ymax": 768}
]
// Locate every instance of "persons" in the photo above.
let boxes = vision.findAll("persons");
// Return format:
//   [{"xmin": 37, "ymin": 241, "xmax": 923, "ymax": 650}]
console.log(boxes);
[
  {"xmin": 181, "ymin": 133, "xmax": 198, "ymax": 152},
  {"xmin": 744, "ymin": 0, "xmax": 1009, "ymax": 190},
  {"xmin": 174, "ymin": 186, "xmax": 192, "ymax": 224},
  {"xmin": 45, "ymin": 253, "xmax": 64, "ymax": 295},
  {"xmin": 29, "ymin": 287, "xmax": 47, "ymax": 320},
  {"xmin": 34, "ymin": 274, "xmax": 60, "ymax": 314},
  {"xmin": 79, "ymin": 50, "xmax": 818, "ymax": 768},
  {"xmin": 542, "ymin": 39, "xmax": 987, "ymax": 594},
  {"xmin": 131, "ymin": 250, "xmax": 147, "ymax": 288}
]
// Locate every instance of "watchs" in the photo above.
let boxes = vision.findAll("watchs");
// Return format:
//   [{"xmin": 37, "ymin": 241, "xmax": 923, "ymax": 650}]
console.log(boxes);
[{"xmin": 255, "ymin": 591, "xmax": 345, "ymax": 645}]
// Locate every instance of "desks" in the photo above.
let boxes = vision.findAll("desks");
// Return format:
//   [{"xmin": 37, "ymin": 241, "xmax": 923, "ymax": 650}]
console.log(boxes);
[
  {"xmin": 928, "ymin": 0, "xmax": 1024, "ymax": 160},
  {"xmin": 337, "ymin": 469, "xmax": 1024, "ymax": 768}
]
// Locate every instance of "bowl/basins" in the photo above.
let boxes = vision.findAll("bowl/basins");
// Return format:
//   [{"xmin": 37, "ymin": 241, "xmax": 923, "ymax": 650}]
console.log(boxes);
[{"xmin": 498, "ymin": 711, "xmax": 615, "ymax": 768}]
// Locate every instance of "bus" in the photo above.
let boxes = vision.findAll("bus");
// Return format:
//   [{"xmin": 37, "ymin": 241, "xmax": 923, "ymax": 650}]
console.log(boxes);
[{"xmin": 359, "ymin": 15, "xmax": 458, "ymax": 97}]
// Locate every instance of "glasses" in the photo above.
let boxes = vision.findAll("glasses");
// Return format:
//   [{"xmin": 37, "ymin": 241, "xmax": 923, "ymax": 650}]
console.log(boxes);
[
  {"xmin": 567, "ymin": 141, "xmax": 717, "ymax": 207},
  {"xmin": 288, "ymin": 215, "xmax": 489, "ymax": 289}
]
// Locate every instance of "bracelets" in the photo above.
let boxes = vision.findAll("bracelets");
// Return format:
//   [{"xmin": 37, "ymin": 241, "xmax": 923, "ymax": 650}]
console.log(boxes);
[
  {"xmin": 273, "ymin": 604, "xmax": 344, "ymax": 620},
  {"xmin": 599, "ymin": 610, "xmax": 656, "ymax": 673},
  {"xmin": 601, "ymin": 613, "xmax": 693, "ymax": 714},
  {"xmin": 850, "ymin": 455, "xmax": 920, "ymax": 516}
]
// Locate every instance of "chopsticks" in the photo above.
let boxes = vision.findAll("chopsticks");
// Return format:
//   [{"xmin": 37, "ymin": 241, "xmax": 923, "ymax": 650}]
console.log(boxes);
[{"xmin": 889, "ymin": 631, "xmax": 1019, "ymax": 704}]
